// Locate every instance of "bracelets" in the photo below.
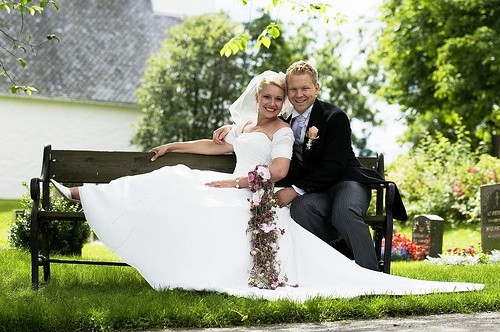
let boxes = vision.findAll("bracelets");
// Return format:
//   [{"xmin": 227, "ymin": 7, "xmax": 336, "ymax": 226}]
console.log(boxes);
[{"xmin": 236, "ymin": 178, "xmax": 240, "ymax": 189}]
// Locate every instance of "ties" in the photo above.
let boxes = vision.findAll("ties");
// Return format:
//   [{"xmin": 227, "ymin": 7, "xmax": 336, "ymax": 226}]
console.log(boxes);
[{"xmin": 293, "ymin": 115, "xmax": 305, "ymax": 166}]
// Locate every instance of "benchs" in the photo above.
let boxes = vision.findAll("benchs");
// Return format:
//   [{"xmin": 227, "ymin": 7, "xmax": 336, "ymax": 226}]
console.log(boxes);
[{"xmin": 30, "ymin": 145, "xmax": 396, "ymax": 290}]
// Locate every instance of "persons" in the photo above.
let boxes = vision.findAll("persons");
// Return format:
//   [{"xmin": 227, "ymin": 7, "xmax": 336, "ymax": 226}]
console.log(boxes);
[
  {"xmin": 49, "ymin": 71, "xmax": 485, "ymax": 302},
  {"xmin": 214, "ymin": 61, "xmax": 409, "ymax": 273}
]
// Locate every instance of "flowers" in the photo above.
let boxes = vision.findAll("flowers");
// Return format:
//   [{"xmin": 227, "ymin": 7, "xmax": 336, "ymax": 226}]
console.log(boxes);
[
  {"xmin": 246, "ymin": 165, "xmax": 299, "ymax": 289},
  {"xmin": 305, "ymin": 126, "xmax": 320, "ymax": 150}
]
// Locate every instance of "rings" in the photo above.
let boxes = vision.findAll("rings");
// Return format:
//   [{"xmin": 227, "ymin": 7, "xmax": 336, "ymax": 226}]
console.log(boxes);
[{"xmin": 213, "ymin": 131, "xmax": 216, "ymax": 134}]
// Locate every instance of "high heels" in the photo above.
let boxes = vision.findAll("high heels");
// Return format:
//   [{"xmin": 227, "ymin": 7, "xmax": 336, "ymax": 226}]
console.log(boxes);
[{"xmin": 50, "ymin": 179, "xmax": 83, "ymax": 212}]
